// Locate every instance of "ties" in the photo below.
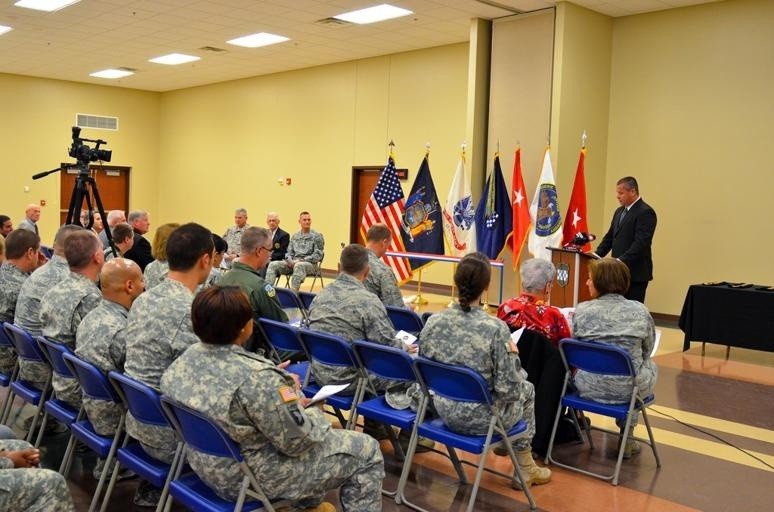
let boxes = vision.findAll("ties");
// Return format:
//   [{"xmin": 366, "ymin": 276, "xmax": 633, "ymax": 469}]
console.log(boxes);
[{"xmin": 619, "ymin": 208, "xmax": 627, "ymax": 222}]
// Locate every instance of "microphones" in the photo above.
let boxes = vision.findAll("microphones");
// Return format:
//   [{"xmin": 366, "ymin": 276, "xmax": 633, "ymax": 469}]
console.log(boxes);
[
  {"xmin": 562, "ymin": 232, "xmax": 586, "ymax": 248},
  {"xmin": 573, "ymin": 233, "xmax": 596, "ymax": 246}
]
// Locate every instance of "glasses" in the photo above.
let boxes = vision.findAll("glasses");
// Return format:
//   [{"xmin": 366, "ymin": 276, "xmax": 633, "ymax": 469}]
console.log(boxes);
[{"xmin": 262, "ymin": 246, "xmax": 274, "ymax": 252}]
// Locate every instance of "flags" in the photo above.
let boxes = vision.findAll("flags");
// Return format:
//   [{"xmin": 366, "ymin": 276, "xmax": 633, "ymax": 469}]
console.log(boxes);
[
  {"xmin": 401, "ymin": 157, "xmax": 443, "ymax": 273},
  {"xmin": 563, "ymin": 150, "xmax": 593, "ymax": 253},
  {"xmin": 507, "ymin": 148, "xmax": 532, "ymax": 272},
  {"xmin": 477, "ymin": 153, "xmax": 513, "ymax": 263},
  {"xmin": 444, "ymin": 157, "xmax": 476, "ymax": 254},
  {"xmin": 528, "ymin": 151, "xmax": 564, "ymax": 263},
  {"xmin": 361, "ymin": 157, "xmax": 412, "ymax": 291}
]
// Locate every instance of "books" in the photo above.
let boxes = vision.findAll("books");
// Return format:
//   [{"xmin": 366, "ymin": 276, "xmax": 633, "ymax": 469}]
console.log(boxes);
[{"xmin": 401, "ymin": 295, "xmax": 416, "ymax": 305}]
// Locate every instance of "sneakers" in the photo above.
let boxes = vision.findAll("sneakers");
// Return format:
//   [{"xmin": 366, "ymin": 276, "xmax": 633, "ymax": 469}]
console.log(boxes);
[{"xmin": 577, "ymin": 416, "xmax": 591, "ymax": 430}]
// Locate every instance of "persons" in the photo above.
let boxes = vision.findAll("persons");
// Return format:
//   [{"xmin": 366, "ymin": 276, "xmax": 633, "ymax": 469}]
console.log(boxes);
[
  {"xmin": 593, "ymin": 177, "xmax": 655, "ymax": 306},
  {"xmin": 418, "ymin": 250, "xmax": 552, "ymax": 491},
  {"xmin": 495, "ymin": 256, "xmax": 591, "ymax": 432},
  {"xmin": 158, "ymin": 283, "xmax": 386, "ymax": 510},
  {"xmin": 298, "ymin": 243, "xmax": 437, "ymax": 456},
  {"xmin": 0, "ymin": 202, "xmax": 326, "ymax": 512},
  {"xmin": 571, "ymin": 257, "xmax": 659, "ymax": 459},
  {"xmin": 355, "ymin": 223, "xmax": 417, "ymax": 316}
]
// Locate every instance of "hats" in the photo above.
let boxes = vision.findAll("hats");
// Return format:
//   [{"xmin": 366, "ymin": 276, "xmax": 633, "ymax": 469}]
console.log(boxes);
[
  {"xmin": 92, "ymin": 456, "xmax": 136, "ymax": 481},
  {"xmin": 23, "ymin": 413, "xmax": 68, "ymax": 433},
  {"xmin": 385, "ymin": 380, "xmax": 417, "ymax": 410},
  {"xmin": 133, "ymin": 479, "xmax": 160, "ymax": 507}
]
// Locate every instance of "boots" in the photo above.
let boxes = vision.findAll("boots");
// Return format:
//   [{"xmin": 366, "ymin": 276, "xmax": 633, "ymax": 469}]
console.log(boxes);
[
  {"xmin": 398, "ymin": 429, "xmax": 435, "ymax": 454},
  {"xmin": 363, "ymin": 416, "xmax": 400, "ymax": 440},
  {"xmin": 512, "ymin": 447, "xmax": 552, "ymax": 490},
  {"xmin": 618, "ymin": 427, "xmax": 641, "ymax": 459}
]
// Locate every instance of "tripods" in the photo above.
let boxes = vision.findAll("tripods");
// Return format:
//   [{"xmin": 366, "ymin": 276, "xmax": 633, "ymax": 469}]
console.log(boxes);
[{"xmin": 63, "ymin": 175, "xmax": 119, "ymax": 260}]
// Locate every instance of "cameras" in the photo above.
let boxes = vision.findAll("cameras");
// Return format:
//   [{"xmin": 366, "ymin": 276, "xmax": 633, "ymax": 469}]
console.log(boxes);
[{"xmin": 69, "ymin": 126, "xmax": 112, "ymax": 163}]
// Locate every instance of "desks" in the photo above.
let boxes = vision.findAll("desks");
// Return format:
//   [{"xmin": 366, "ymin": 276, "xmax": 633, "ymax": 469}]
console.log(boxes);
[{"xmin": 689, "ymin": 282, "xmax": 774, "ymax": 359}]
